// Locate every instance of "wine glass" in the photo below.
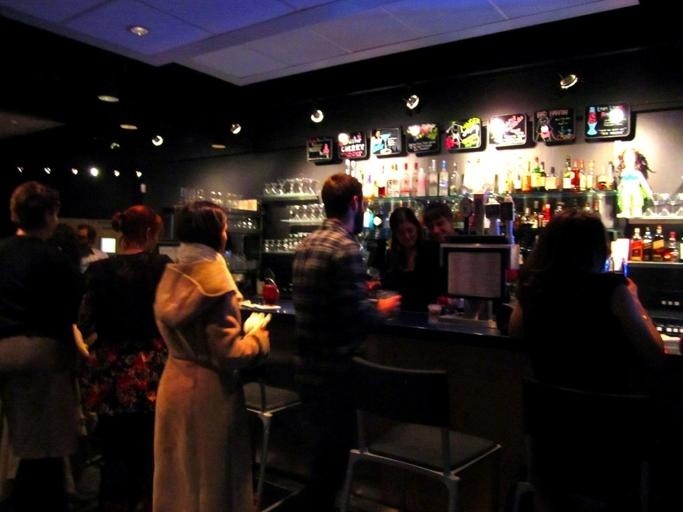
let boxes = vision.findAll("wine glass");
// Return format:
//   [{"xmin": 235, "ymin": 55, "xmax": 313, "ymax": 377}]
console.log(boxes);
[
  {"xmin": 262, "ymin": 232, "xmax": 310, "ymax": 251},
  {"xmin": 262, "ymin": 177, "xmax": 326, "ymax": 220},
  {"xmin": 186, "ymin": 189, "xmax": 256, "ymax": 229}
]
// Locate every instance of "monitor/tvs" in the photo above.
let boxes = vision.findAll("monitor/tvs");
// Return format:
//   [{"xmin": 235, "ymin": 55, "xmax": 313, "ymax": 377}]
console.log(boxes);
[
  {"xmin": 439, "ymin": 243, "xmax": 512, "ymax": 320},
  {"xmin": 627, "ymin": 261, "xmax": 683, "ymax": 326}
]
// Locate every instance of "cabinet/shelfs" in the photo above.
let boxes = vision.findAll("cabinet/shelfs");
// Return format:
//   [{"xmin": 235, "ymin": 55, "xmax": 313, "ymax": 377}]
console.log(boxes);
[
  {"xmin": 264, "ymin": 194, "xmax": 324, "ymax": 256},
  {"xmin": 227, "ymin": 208, "xmax": 262, "ymax": 274},
  {"xmin": 621, "ymin": 216, "xmax": 682, "ymax": 270}
]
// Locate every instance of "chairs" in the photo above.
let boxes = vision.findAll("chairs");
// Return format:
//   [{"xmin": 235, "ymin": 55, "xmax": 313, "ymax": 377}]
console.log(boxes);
[
  {"xmin": 521, "ymin": 373, "xmax": 658, "ymax": 512},
  {"xmin": 338, "ymin": 356, "xmax": 504, "ymax": 511},
  {"xmin": 234, "ymin": 364, "xmax": 309, "ymax": 511}
]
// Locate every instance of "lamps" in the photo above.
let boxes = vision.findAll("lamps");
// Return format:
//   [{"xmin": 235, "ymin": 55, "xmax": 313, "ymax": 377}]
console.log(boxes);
[{"xmin": 149, "ymin": 64, "xmax": 578, "ymax": 148}]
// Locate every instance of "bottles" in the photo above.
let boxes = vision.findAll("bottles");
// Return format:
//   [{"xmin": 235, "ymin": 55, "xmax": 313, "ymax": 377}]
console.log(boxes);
[
  {"xmin": 372, "ymin": 201, "xmax": 464, "ymax": 239},
  {"xmin": 341, "ymin": 156, "xmax": 463, "ymax": 195},
  {"xmin": 585, "ymin": 107, "xmax": 598, "ymax": 135},
  {"xmin": 628, "ymin": 223, "xmax": 678, "ymax": 263},
  {"xmin": 505, "ymin": 153, "xmax": 595, "ymax": 194},
  {"xmin": 513, "ymin": 198, "xmax": 592, "ymax": 229}
]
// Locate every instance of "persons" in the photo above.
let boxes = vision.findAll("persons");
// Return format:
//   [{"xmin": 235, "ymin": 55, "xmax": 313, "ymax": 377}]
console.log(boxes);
[
  {"xmin": 151, "ymin": 200, "xmax": 271, "ymax": 509},
  {"xmin": 3, "ymin": 181, "xmax": 106, "ymax": 511},
  {"xmin": 80, "ymin": 204, "xmax": 176, "ymax": 509},
  {"xmin": 510, "ymin": 211, "xmax": 665, "ymax": 509},
  {"xmin": 382, "ymin": 202, "xmax": 457, "ymax": 312},
  {"xmin": 291, "ymin": 172, "xmax": 401, "ymax": 509}
]
